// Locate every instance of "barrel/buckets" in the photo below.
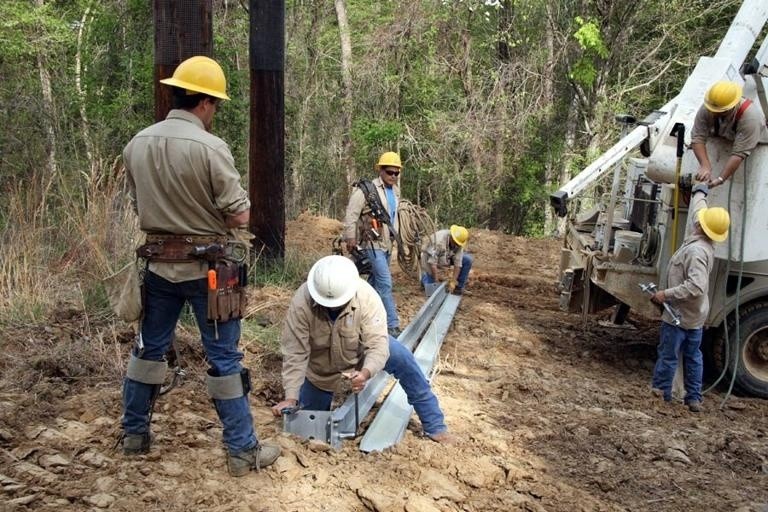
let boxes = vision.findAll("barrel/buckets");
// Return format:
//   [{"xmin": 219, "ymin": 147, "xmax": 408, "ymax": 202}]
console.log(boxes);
[{"xmin": 612, "ymin": 229, "xmax": 643, "ymax": 262}]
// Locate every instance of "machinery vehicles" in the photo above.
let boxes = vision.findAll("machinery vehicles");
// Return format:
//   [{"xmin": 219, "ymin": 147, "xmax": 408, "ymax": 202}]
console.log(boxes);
[{"xmin": 549, "ymin": 0, "xmax": 768, "ymax": 396}]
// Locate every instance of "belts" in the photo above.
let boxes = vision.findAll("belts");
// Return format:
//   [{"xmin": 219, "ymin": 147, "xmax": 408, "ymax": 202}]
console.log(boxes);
[{"xmin": 136, "ymin": 233, "xmax": 233, "ymax": 264}]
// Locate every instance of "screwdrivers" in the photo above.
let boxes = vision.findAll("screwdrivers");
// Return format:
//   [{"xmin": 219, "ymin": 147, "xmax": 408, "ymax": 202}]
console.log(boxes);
[
  {"xmin": 208, "ymin": 270, "xmax": 219, "ymax": 341},
  {"xmin": 372, "ymin": 219, "xmax": 382, "ymax": 249}
]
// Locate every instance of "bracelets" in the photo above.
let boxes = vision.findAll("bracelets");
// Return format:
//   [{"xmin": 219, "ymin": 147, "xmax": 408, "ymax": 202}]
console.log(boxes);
[{"xmin": 718, "ymin": 176, "xmax": 724, "ymax": 184}]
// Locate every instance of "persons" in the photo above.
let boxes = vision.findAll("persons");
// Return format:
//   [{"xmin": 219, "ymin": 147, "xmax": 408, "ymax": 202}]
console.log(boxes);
[
  {"xmin": 415, "ymin": 224, "xmax": 474, "ymax": 299},
  {"xmin": 119, "ymin": 56, "xmax": 284, "ymax": 477},
  {"xmin": 269, "ymin": 252, "xmax": 452, "ymax": 445},
  {"xmin": 647, "ymin": 172, "xmax": 733, "ymax": 416},
  {"xmin": 341, "ymin": 151, "xmax": 402, "ymax": 341},
  {"xmin": 691, "ymin": 80, "xmax": 768, "ymax": 188}
]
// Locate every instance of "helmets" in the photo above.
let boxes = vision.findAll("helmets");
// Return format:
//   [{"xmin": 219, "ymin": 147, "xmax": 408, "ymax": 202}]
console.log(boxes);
[
  {"xmin": 307, "ymin": 255, "xmax": 360, "ymax": 309},
  {"xmin": 704, "ymin": 81, "xmax": 743, "ymax": 112},
  {"xmin": 450, "ymin": 224, "xmax": 469, "ymax": 245},
  {"xmin": 376, "ymin": 151, "xmax": 402, "ymax": 169},
  {"xmin": 159, "ymin": 56, "xmax": 232, "ymax": 102},
  {"xmin": 698, "ymin": 207, "xmax": 731, "ymax": 242}
]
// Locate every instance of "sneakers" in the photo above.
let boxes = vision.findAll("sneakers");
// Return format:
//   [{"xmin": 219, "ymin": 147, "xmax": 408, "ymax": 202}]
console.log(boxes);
[
  {"xmin": 689, "ymin": 401, "xmax": 699, "ymax": 411},
  {"xmin": 429, "ymin": 431, "xmax": 458, "ymax": 446},
  {"xmin": 123, "ymin": 432, "xmax": 154, "ymax": 455},
  {"xmin": 227, "ymin": 441, "xmax": 281, "ymax": 476},
  {"xmin": 388, "ymin": 327, "xmax": 402, "ymax": 339},
  {"xmin": 453, "ymin": 286, "xmax": 473, "ymax": 297}
]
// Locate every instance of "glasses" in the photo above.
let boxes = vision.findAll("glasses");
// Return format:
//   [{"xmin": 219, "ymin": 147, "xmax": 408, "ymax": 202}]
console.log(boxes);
[{"xmin": 382, "ymin": 168, "xmax": 399, "ymax": 175}]
